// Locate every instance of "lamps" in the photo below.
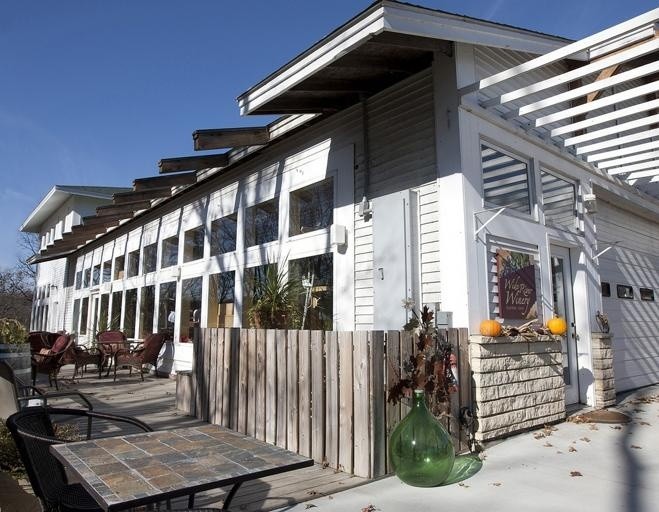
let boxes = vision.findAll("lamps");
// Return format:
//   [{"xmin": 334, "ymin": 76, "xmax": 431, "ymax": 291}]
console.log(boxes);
[
  {"xmin": 583, "ymin": 188, "xmax": 597, "ymax": 214},
  {"xmin": 330, "ymin": 224, "xmax": 346, "ymax": 246}
]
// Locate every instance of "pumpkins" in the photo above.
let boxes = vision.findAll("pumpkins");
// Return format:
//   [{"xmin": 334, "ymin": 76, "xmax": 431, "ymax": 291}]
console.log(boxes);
[
  {"xmin": 546, "ymin": 317, "xmax": 567, "ymax": 335},
  {"xmin": 480, "ymin": 320, "xmax": 501, "ymax": 337}
]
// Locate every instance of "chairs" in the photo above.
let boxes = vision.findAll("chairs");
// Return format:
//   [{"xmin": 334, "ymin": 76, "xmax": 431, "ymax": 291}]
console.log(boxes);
[
  {"xmin": 29, "ymin": 331, "xmax": 168, "ymax": 394},
  {"xmin": 388, "ymin": 298, "xmax": 457, "ymax": 404},
  {"xmin": 0, "ymin": 358, "xmax": 93, "ymax": 440},
  {"xmin": 7, "ymin": 407, "xmax": 154, "ymax": 512},
  {"xmin": 49, "ymin": 424, "xmax": 315, "ymax": 512},
  {"xmin": 389, "ymin": 389, "xmax": 456, "ymax": 488}
]
